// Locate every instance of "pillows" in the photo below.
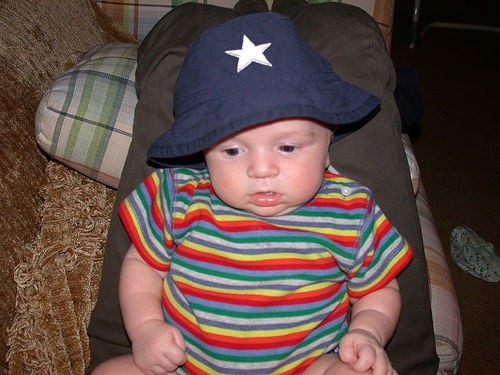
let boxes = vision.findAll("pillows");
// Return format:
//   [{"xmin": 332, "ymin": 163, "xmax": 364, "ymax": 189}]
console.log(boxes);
[{"xmin": 33, "ymin": 38, "xmax": 139, "ymax": 188}]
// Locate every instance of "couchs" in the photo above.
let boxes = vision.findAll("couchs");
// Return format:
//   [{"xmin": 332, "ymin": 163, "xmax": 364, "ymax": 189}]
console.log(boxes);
[{"xmin": 0, "ymin": 0, "xmax": 465, "ymax": 375}]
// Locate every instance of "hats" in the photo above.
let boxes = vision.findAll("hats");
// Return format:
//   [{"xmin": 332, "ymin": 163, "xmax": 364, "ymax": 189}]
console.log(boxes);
[{"xmin": 148, "ymin": 12, "xmax": 382, "ymax": 170}]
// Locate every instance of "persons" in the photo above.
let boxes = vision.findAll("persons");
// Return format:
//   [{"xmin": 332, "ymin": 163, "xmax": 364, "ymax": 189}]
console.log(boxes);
[
  {"xmin": 88, "ymin": 0, "xmax": 445, "ymax": 375},
  {"xmin": 87, "ymin": 9, "xmax": 415, "ymax": 374}
]
